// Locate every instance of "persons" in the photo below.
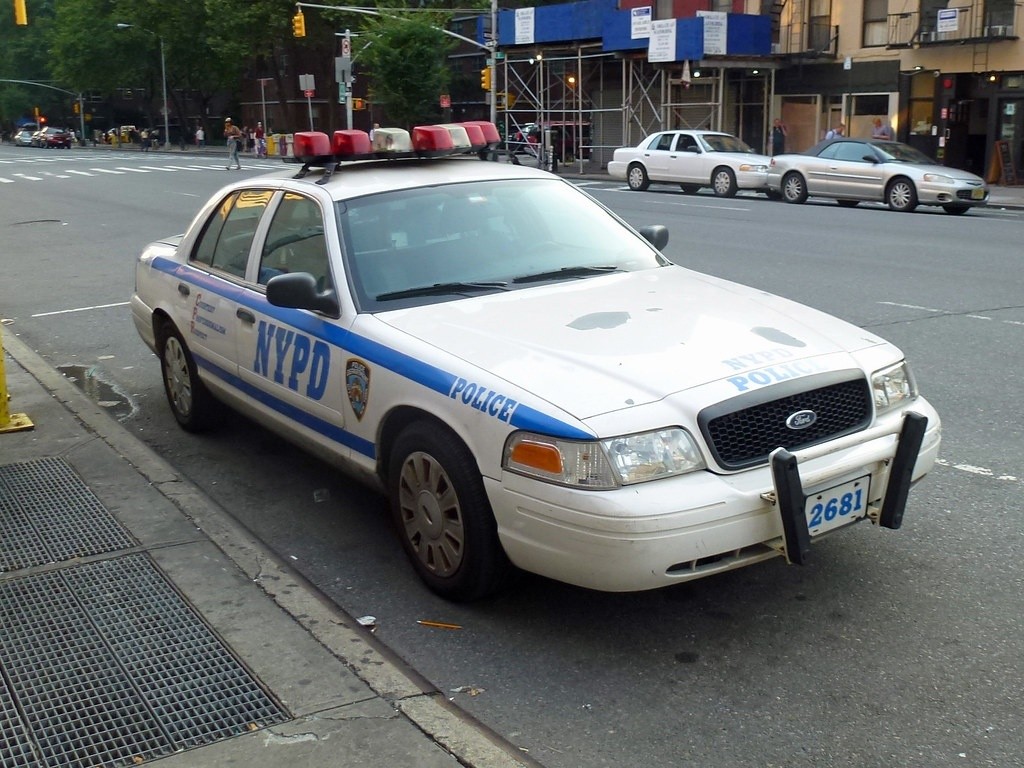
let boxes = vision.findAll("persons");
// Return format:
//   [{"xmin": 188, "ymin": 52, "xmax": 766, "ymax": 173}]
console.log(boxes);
[
  {"xmin": 224, "ymin": 117, "xmax": 242, "ymax": 170},
  {"xmin": 369, "ymin": 123, "xmax": 384, "ymax": 142},
  {"xmin": 826, "ymin": 123, "xmax": 845, "ymax": 140},
  {"xmin": 194, "ymin": 125, "xmax": 205, "ymax": 148},
  {"xmin": 97, "ymin": 126, "xmax": 159, "ymax": 152},
  {"xmin": 238, "ymin": 121, "xmax": 273, "ymax": 156},
  {"xmin": 872, "ymin": 117, "xmax": 890, "ymax": 140},
  {"xmin": 66, "ymin": 128, "xmax": 81, "ymax": 142},
  {"xmin": 769, "ymin": 118, "xmax": 787, "ymax": 155}
]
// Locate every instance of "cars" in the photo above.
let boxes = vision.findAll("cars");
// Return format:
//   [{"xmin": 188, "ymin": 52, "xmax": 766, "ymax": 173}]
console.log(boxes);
[
  {"xmin": 608, "ymin": 129, "xmax": 773, "ymax": 198},
  {"xmin": 766, "ymin": 137, "xmax": 990, "ymax": 215},
  {"xmin": 14, "ymin": 128, "xmax": 72, "ymax": 149},
  {"xmin": 131, "ymin": 120, "xmax": 940, "ymax": 605},
  {"xmin": 497, "ymin": 120, "xmax": 590, "ymax": 159}
]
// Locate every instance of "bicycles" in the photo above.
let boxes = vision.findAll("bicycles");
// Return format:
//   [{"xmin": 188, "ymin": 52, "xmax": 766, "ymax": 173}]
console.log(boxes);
[{"xmin": 178, "ymin": 136, "xmax": 189, "ymax": 150}]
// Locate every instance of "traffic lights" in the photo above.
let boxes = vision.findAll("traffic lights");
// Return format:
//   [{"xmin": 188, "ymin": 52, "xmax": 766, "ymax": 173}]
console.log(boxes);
[
  {"xmin": 356, "ymin": 99, "xmax": 365, "ymax": 110},
  {"xmin": 481, "ymin": 68, "xmax": 490, "ymax": 90},
  {"xmin": 40, "ymin": 117, "xmax": 46, "ymax": 122},
  {"xmin": 509, "ymin": 95, "xmax": 516, "ymax": 106},
  {"xmin": 293, "ymin": 14, "xmax": 306, "ymax": 37},
  {"xmin": 85, "ymin": 115, "xmax": 92, "ymax": 120},
  {"xmin": 74, "ymin": 103, "xmax": 79, "ymax": 113}
]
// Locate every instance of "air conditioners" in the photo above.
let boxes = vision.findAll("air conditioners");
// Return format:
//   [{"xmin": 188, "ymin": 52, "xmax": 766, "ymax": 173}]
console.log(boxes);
[
  {"xmin": 919, "ymin": 31, "xmax": 936, "ymax": 42},
  {"xmin": 984, "ymin": 26, "xmax": 1006, "ymax": 36}
]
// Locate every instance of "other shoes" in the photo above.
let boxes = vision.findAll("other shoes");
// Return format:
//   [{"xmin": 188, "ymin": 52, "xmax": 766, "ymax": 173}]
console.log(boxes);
[
  {"xmin": 236, "ymin": 166, "xmax": 241, "ymax": 169},
  {"xmin": 226, "ymin": 166, "xmax": 230, "ymax": 170}
]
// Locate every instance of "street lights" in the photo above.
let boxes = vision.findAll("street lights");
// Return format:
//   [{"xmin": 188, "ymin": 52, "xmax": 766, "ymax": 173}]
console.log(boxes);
[
  {"xmin": 535, "ymin": 55, "xmax": 543, "ymax": 141},
  {"xmin": 569, "ymin": 76, "xmax": 576, "ymax": 161},
  {"xmin": 117, "ymin": 23, "xmax": 172, "ymax": 148}
]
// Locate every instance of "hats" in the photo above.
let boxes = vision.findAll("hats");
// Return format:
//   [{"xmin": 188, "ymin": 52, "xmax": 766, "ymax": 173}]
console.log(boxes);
[
  {"xmin": 258, "ymin": 125, "xmax": 263, "ymax": 127},
  {"xmin": 225, "ymin": 118, "xmax": 232, "ymax": 121}
]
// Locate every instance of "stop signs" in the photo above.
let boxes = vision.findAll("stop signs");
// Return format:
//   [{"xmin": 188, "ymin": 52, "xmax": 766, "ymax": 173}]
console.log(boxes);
[{"xmin": 304, "ymin": 90, "xmax": 314, "ymax": 98}]
